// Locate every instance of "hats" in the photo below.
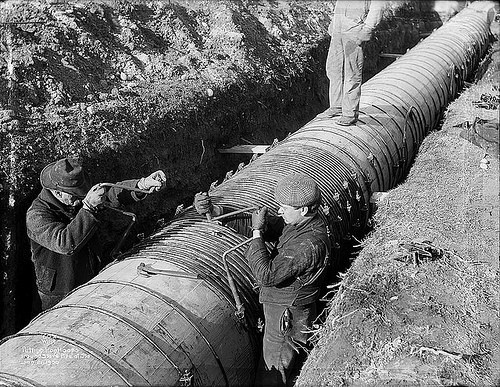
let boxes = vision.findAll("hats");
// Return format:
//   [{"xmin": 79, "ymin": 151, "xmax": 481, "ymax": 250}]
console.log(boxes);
[
  {"xmin": 40, "ymin": 158, "xmax": 92, "ymax": 199},
  {"xmin": 275, "ymin": 173, "xmax": 320, "ymax": 207}
]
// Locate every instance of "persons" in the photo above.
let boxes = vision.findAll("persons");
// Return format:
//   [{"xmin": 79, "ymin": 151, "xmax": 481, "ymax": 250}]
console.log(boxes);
[
  {"xmin": 192, "ymin": 174, "xmax": 333, "ymax": 387},
  {"xmin": 26, "ymin": 157, "xmax": 167, "ymax": 310},
  {"xmin": 323, "ymin": 0, "xmax": 382, "ymax": 125}
]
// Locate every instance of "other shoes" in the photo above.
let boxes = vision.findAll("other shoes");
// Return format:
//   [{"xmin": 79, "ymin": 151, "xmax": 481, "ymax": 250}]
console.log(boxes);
[
  {"xmin": 324, "ymin": 107, "xmax": 343, "ymax": 118},
  {"xmin": 336, "ymin": 116, "xmax": 358, "ymax": 126}
]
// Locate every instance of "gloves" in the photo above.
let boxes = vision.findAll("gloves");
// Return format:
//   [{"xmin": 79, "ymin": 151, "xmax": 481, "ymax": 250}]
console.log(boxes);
[
  {"xmin": 356, "ymin": 30, "xmax": 372, "ymax": 46},
  {"xmin": 252, "ymin": 206, "xmax": 269, "ymax": 233},
  {"xmin": 137, "ymin": 170, "xmax": 167, "ymax": 192},
  {"xmin": 85, "ymin": 183, "xmax": 106, "ymax": 210},
  {"xmin": 194, "ymin": 191, "xmax": 212, "ymax": 216}
]
